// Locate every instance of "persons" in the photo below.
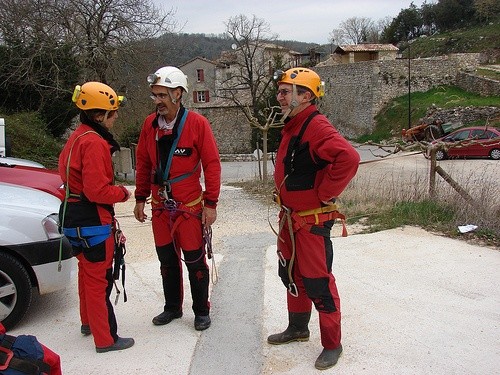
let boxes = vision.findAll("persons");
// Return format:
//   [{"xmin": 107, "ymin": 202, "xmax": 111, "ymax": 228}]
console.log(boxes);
[
  {"xmin": 267, "ymin": 68, "xmax": 360, "ymax": 369},
  {"xmin": 133, "ymin": 67, "xmax": 221, "ymax": 330},
  {"xmin": 59, "ymin": 82, "xmax": 134, "ymax": 353}
]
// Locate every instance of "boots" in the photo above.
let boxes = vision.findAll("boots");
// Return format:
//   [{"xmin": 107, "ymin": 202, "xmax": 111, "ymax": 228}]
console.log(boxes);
[
  {"xmin": 267, "ymin": 312, "xmax": 311, "ymax": 345},
  {"xmin": 314, "ymin": 345, "xmax": 343, "ymax": 370}
]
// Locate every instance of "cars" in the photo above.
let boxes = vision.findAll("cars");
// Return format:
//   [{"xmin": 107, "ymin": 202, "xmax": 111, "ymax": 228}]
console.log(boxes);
[
  {"xmin": 0, "ymin": 157, "xmax": 73, "ymax": 329},
  {"xmin": 426, "ymin": 127, "xmax": 500, "ymax": 160}
]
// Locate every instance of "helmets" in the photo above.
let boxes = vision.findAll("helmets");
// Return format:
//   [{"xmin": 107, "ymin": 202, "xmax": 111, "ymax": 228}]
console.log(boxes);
[
  {"xmin": 72, "ymin": 82, "xmax": 125, "ymax": 111},
  {"xmin": 273, "ymin": 67, "xmax": 325, "ymax": 99},
  {"xmin": 146, "ymin": 66, "xmax": 189, "ymax": 93}
]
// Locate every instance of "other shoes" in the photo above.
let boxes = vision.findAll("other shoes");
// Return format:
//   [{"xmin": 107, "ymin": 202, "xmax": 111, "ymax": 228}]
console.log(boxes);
[
  {"xmin": 79, "ymin": 323, "xmax": 93, "ymax": 335},
  {"xmin": 96, "ymin": 337, "xmax": 134, "ymax": 353},
  {"xmin": 194, "ymin": 314, "xmax": 211, "ymax": 330},
  {"xmin": 152, "ymin": 306, "xmax": 183, "ymax": 325}
]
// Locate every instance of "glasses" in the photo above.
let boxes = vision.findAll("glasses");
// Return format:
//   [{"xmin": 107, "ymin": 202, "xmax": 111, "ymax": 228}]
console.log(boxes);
[
  {"xmin": 274, "ymin": 89, "xmax": 302, "ymax": 97},
  {"xmin": 150, "ymin": 93, "xmax": 178, "ymax": 100}
]
